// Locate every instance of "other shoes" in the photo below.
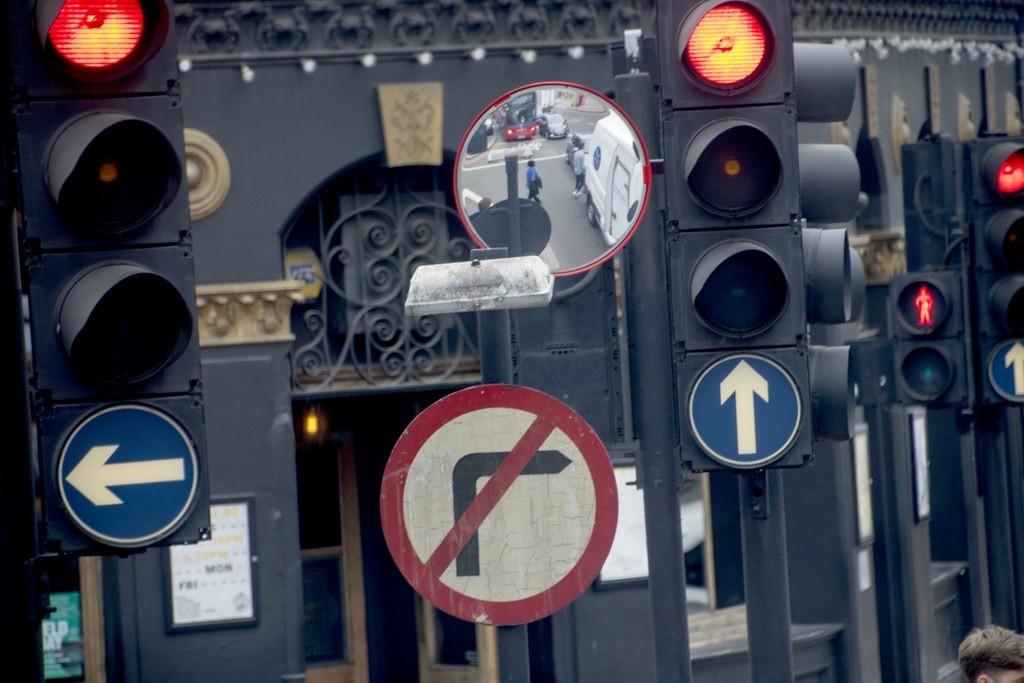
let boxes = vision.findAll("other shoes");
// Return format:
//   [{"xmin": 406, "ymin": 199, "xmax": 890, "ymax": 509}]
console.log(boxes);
[{"xmin": 573, "ymin": 191, "xmax": 581, "ymax": 198}]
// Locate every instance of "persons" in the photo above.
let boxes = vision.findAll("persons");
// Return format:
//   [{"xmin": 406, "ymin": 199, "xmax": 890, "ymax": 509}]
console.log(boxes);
[
  {"xmin": 959, "ymin": 624, "xmax": 1024, "ymax": 683},
  {"xmin": 525, "ymin": 141, "xmax": 588, "ymax": 204}
]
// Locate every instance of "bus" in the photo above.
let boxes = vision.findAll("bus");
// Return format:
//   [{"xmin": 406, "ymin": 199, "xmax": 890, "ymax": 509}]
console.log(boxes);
[{"xmin": 494, "ymin": 90, "xmax": 540, "ymax": 143}]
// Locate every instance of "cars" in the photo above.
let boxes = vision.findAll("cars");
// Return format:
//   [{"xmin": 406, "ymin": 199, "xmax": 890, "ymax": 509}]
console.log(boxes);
[{"xmin": 538, "ymin": 113, "xmax": 570, "ymax": 139}]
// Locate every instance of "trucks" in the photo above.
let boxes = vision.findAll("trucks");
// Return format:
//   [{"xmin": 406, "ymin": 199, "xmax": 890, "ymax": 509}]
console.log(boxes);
[{"xmin": 582, "ymin": 109, "xmax": 647, "ymax": 248}]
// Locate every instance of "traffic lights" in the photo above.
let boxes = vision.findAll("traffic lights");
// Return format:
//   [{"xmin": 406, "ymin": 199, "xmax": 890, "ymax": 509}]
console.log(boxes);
[
  {"xmin": 890, "ymin": 269, "xmax": 969, "ymax": 407},
  {"xmin": 657, "ymin": 0, "xmax": 815, "ymax": 474},
  {"xmin": 793, "ymin": 40, "xmax": 864, "ymax": 442},
  {"xmin": 0, "ymin": 1, "xmax": 213, "ymax": 566},
  {"xmin": 964, "ymin": 137, "xmax": 1024, "ymax": 405}
]
